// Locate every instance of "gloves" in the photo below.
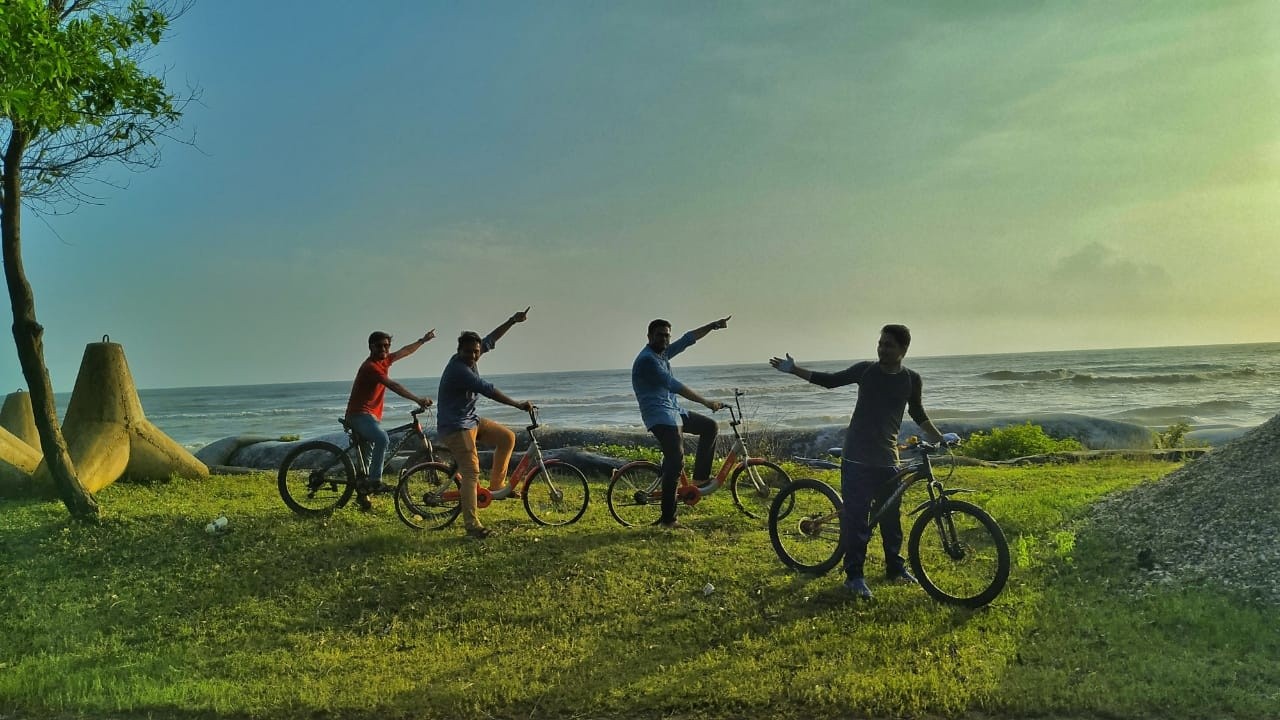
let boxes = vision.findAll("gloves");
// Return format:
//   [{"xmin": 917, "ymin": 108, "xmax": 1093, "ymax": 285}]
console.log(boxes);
[{"xmin": 942, "ymin": 433, "xmax": 961, "ymax": 449}]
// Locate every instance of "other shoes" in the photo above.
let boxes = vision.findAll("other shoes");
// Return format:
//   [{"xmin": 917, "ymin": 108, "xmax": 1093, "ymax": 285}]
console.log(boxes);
[
  {"xmin": 369, "ymin": 481, "xmax": 396, "ymax": 493},
  {"xmin": 850, "ymin": 583, "xmax": 871, "ymax": 599},
  {"xmin": 696, "ymin": 481, "xmax": 711, "ymax": 488},
  {"xmin": 887, "ymin": 571, "xmax": 918, "ymax": 585},
  {"xmin": 356, "ymin": 495, "xmax": 371, "ymax": 509},
  {"xmin": 487, "ymin": 487, "xmax": 520, "ymax": 499},
  {"xmin": 466, "ymin": 528, "xmax": 500, "ymax": 538},
  {"xmin": 662, "ymin": 522, "xmax": 688, "ymax": 529}
]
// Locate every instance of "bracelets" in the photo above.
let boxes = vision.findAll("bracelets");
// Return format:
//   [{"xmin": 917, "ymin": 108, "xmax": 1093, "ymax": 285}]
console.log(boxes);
[
  {"xmin": 419, "ymin": 339, "xmax": 424, "ymax": 344},
  {"xmin": 713, "ymin": 321, "xmax": 720, "ymax": 330},
  {"xmin": 509, "ymin": 317, "xmax": 515, "ymax": 325}
]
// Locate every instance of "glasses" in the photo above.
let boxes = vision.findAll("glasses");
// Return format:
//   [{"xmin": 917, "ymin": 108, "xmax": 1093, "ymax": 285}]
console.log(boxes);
[
  {"xmin": 652, "ymin": 332, "xmax": 672, "ymax": 339},
  {"xmin": 375, "ymin": 343, "xmax": 391, "ymax": 350}
]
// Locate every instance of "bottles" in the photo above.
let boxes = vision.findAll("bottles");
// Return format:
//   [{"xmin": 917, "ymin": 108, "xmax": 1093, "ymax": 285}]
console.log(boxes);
[{"xmin": 205, "ymin": 517, "xmax": 228, "ymax": 532}]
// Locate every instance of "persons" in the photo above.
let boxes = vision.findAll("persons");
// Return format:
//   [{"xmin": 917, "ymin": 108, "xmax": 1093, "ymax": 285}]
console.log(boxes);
[
  {"xmin": 633, "ymin": 314, "xmax": 732, "ymax": 529},
  {"xmin": 436, "ymin": 305, "xmax": 534, "ymax": 540},
  {"xmin": 768, "ymin": 324, "xmax": 961, "ymax": 602},
  {"xmin": 344, "ymin": 328, "xmax": 437, "ymax": 510}
]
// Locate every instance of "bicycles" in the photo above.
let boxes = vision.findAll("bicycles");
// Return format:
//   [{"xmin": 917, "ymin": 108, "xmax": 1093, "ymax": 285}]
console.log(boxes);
[
  {"xmin": 392, "ymin": 405, "xmax": 591, "ymax": 531},
  {"xmin": 767, "ymin": 432, "xmax": 1011, "ymax": 609},
  {"xmin": 607, "ymin": 388, "xmax": 795, "ymax": 531},
  {"xmin": 276, "ymin": 403, "xmax": 465, "ymax": 521}
]
